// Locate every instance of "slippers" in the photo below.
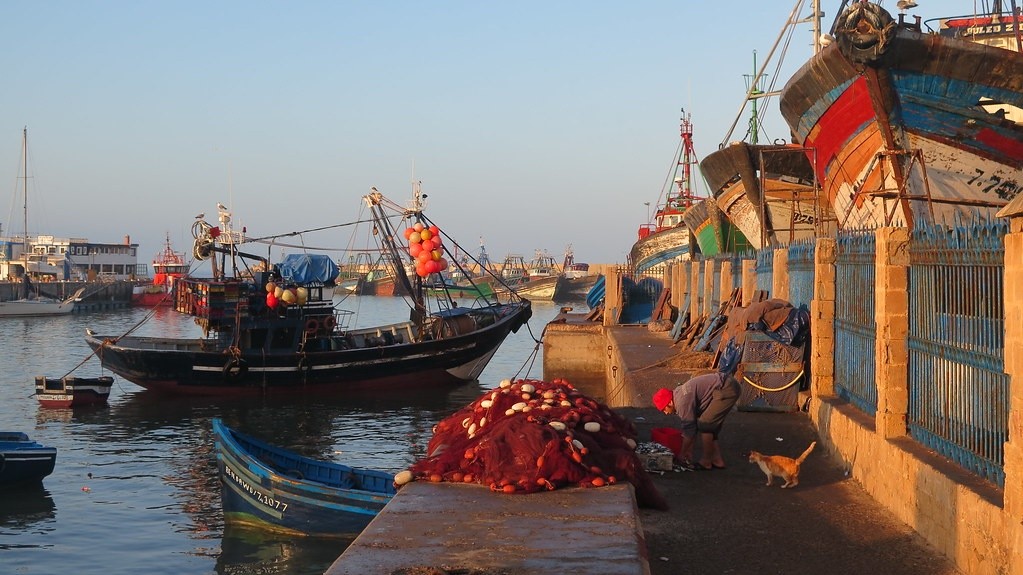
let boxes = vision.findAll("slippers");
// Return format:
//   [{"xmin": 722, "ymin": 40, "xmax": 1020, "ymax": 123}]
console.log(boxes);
[
  {"xmin": 687, "ymin": 462, "xmax": 710, "ymax": 471},
  {"xmin": 710, "ymin": 461, "xmax": 724, "ymax": 469}
]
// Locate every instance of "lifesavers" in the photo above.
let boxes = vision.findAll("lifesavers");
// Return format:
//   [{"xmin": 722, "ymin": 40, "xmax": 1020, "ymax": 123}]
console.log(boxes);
[
  {"xmin": 324, "ymin": 316, "xmax": 336, "ymax": 330},
  {"xmin": 305, "ymin": 319, "xmax": 319, "ymax": 334},
  {"xmin": 835, "ymin": 2, "xmax": 895, "ymax": 64}
]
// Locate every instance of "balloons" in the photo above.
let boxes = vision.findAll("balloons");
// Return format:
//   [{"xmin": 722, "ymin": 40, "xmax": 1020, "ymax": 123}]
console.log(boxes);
[
  {"xmin": 265, "ymin": 281, "xmax": 308, "ymax": 308},
  {"xmin": 405, "ymin": 222, "xmax": 448, "ymax": 276}
]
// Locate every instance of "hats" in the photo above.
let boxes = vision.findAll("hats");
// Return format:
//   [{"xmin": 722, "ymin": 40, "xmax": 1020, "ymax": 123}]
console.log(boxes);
[{"xmin": 652, "ymin": 388, "xmax": 672, "ymax": 412}]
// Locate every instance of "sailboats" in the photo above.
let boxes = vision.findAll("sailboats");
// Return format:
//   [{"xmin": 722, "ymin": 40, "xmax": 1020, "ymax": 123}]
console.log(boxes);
[{"xmin": 0, "ymin": 123, "xmax": 86, "ymax": 319}]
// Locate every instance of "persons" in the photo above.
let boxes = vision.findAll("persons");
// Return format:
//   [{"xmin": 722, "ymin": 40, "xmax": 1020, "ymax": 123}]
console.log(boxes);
[{"xmin": 652, "ymin": 373, "xmax": 741, "ymax": 470}]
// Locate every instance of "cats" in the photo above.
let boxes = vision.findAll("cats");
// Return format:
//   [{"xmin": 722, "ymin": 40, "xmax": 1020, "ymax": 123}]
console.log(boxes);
[{"xmin": 750, "ymin": 442, "xmax": 816, "ymax": 489}]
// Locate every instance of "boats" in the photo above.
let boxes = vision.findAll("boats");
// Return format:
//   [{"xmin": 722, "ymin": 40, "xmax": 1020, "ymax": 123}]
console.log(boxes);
[
  {"xmin": 34, "ymin": 375, "xmax": 115, "ymax": 411},
  {"xmin": 132, "ymin": 231, "xmax": 191, "ymax": 305},
  {"xmin": 0, "ymin": 431, "xmax": 58, "ymax": 486},
  {"xmin": 210, "ymin": 416, "xmax": 406, "ymax": 543},
  {"xmin": 497, "ymin": 249, "xmax": 531, "ymax": 286},
  {"xmin": 625, "ymin": 0, "xmax": 1023, "ymax": 283},
  {"xmin": 585, "ymin": 273, "xmax": 638, "ymax": 315},
  {"xmin": 82, "ymin": 157, "xmax": 534, "ymax": 403},
  {"xmin": 526, "ymin": 248, "xmax": 562, "ymax": 282},
  {"xmin": 334, "ymin": 244, "xmax": 497, "ymax": 298},
  {"xmin": 561, "ymin": 241, "xmax": 589, "ymax": 281}
]
